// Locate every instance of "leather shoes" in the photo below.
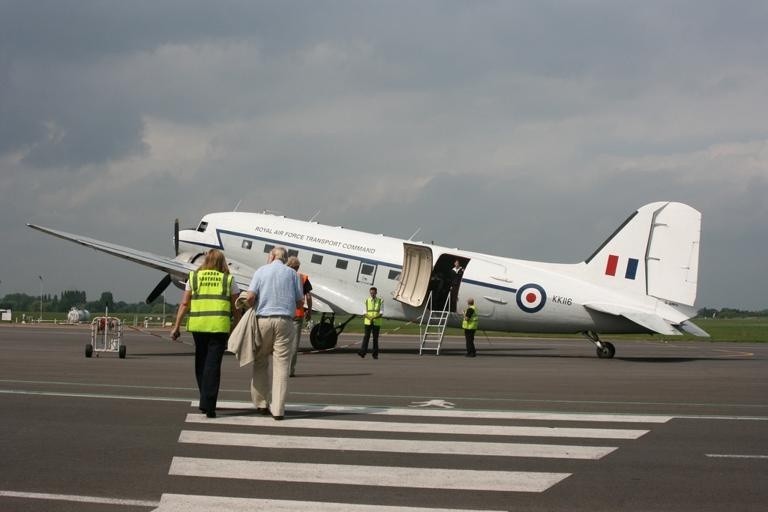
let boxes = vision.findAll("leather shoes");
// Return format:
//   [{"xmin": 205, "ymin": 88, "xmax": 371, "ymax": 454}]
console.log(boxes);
[
  {"xmin": 270, "ymin": 411, "xmax": 283, "ymax": 420},
  {"xmin": 206, "ymin": 412, "xmax": 216, "ymax": 417},
  {"xmin": 198, "ymin": 407, "xmax": 206, "ymax": 414},
  {"xmin": 256, "ymin": 407, "xmax": 268, "ymax": 415}
]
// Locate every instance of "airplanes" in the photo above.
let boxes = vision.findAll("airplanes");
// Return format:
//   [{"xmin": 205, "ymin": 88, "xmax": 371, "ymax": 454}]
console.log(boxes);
[{"xmin": 23, "ymin": 199, "xmax": 715, "ymax": 360}]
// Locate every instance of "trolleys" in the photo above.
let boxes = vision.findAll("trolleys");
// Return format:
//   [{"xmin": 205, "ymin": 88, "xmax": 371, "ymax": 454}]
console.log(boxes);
[{"xmin": 81, "ymin": 300, "xmax": 128, "ymax": 363}]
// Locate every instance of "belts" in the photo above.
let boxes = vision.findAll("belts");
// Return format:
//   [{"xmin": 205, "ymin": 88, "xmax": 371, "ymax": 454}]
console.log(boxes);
[{"xmin": 261, "ymin": 315, "xmax": 291, "ymax": 318}]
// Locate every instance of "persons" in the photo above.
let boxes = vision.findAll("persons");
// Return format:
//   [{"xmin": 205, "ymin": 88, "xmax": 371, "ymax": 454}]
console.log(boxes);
[
  {"xmin": 461, "ymin": 297, "xmax": 481, "ymax": 359},
  {"xmin": 168, "ymin": 248, "xmax": 246, "ymax": 420},
  {"xmin": 242, "ymin": 249, "xmax": 306, "ymax": 420},
  {"xmin": 448, "ymin": 259, "xmax": 464, "ymax": 312},
  {"xmin": 285, "ymin": 256, "xmax": 313, "ymax": 378},
  {"xmin": 357, "ymin": 287, "xmax": 384, "ymax": 359}
]
